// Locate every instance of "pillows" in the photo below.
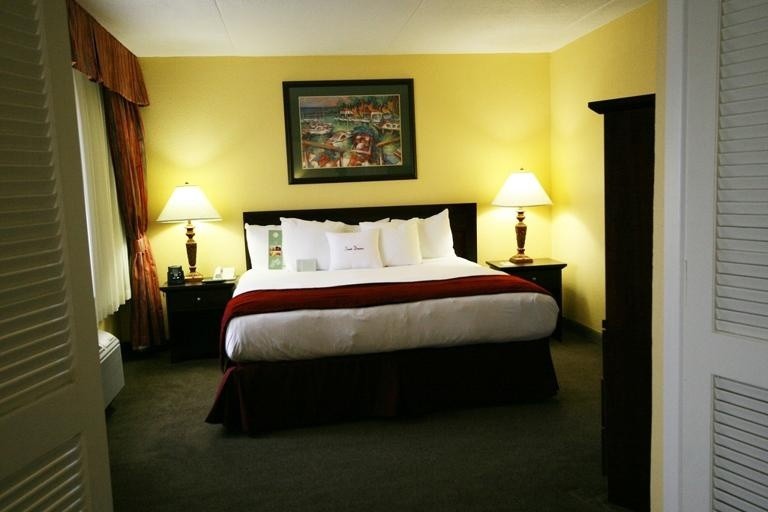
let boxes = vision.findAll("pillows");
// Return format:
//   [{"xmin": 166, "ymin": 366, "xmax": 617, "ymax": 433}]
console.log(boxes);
[
  {"xmin": 411, "ymin": 207, "xmax": 457, "ymax": 260},
  {"xmin": 279, "ymin": 216, "xmax": 347, "ymax": 271},
  {"xmin": 363, "ymin": 220, "xmax": 422, "ymax": 268},
  {"xmin": 242, "ymin": 220, "xmax": 284, "ymax": 270},
  {"xmin": 326, "ymin": 228, "xmax": 381, "ymax": 272}
]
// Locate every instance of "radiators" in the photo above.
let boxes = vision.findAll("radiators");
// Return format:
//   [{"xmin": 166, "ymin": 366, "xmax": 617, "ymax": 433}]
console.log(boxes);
[{"xmin": 96, "ymin": 328, "xmax": 126, "ymax": 410}]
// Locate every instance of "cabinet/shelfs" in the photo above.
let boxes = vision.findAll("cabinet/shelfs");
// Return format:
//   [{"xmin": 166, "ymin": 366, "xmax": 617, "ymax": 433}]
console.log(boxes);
[{"xmin": 588, "ymin": 93, "xmax": 655, "ymax": 510}]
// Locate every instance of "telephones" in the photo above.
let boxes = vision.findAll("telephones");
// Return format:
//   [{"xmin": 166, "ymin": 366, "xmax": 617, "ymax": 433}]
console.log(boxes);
[{"xmin": 213, "ymin": 267, "xmax": 237, "ymax": 281}]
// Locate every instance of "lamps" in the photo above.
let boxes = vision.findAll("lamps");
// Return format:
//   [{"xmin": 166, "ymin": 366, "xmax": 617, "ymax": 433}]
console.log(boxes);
[
  {"xmin": 491, "ymin": 168, "xmax": 555, "ymax": 264},
  {"xmin": 155, "ymin": 182, "xmax": 222, "ymax": 282}
]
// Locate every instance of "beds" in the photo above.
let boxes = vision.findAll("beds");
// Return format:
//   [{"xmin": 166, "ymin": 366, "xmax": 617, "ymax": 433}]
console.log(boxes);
[{"xmin": 204, "ymin": 202, "xmax": 562, "ymax": 434}]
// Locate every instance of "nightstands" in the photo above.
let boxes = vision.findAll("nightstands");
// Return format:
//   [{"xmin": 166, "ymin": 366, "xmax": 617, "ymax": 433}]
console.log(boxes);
[
  {"xmin": 158, "ymin": 275, "xmax": 241, "ymax": 363},
  {"xmin": 485, "ymin": 258, "xmax": 568, "ymax": 326}
]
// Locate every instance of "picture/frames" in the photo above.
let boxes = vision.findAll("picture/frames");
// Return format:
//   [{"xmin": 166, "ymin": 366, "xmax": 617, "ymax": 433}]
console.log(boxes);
[{"xmin": 282, "ymin": 79, "xmax": 417, "ymax": 185}]
